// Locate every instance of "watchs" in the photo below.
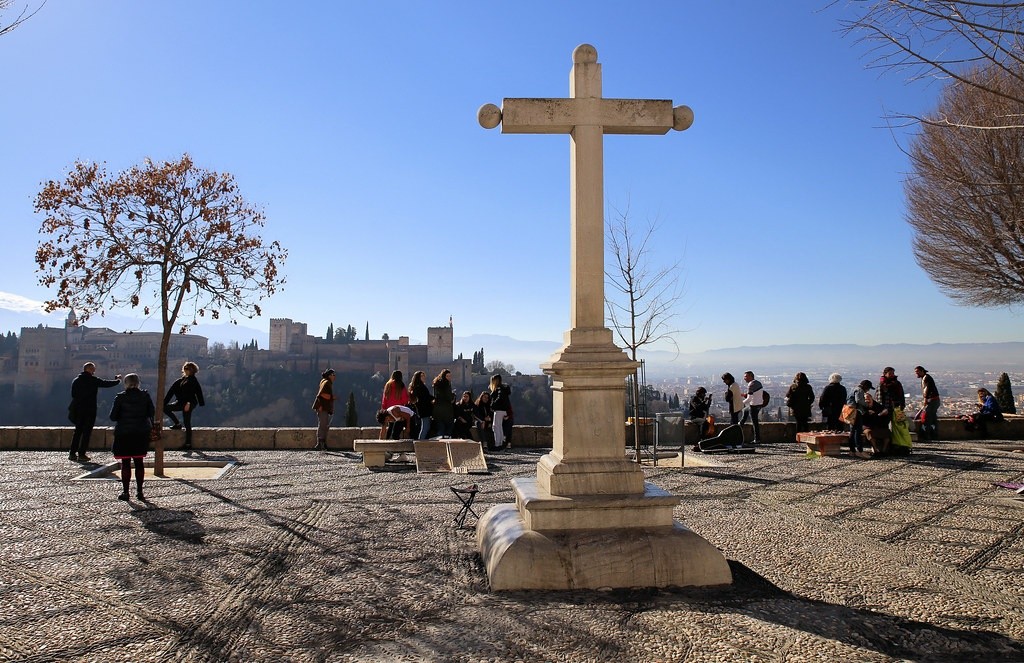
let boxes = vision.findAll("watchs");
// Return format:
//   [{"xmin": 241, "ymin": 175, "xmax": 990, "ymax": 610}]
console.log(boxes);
[{"xmin": 186, "ymin": 402, "xmax": 190, "ymax": 405}]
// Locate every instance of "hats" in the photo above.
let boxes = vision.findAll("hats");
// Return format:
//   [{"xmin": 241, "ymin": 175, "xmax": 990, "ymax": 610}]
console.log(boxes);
[{"xmin": 860, "ymin": 380, "xmax": 876, "ymax": 390}]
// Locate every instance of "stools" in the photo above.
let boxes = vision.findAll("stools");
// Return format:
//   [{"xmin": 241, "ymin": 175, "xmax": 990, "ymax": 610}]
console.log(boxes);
[{"xmin": 450, "ymin": 485, "xmax": 479, "ymax": 529}]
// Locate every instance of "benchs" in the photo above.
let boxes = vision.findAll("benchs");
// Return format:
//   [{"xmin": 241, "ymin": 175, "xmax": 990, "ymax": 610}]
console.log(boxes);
[
  {"xmin": 796, "ymin": 430, "xmax": 918, "ymax": 456},
  {"xmin": 353, "ymin": 439, "xmax": 475, "ymax": 467}
]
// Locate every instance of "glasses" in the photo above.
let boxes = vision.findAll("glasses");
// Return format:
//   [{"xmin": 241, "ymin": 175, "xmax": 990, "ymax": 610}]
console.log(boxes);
[{"xmin": 184, "ymin": 367, "xmax": 188, "ymax": 370}]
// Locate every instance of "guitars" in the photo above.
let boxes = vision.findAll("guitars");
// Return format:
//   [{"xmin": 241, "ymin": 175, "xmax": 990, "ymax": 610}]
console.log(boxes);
[{"xmin": 704, "ymin": 393, "xmax": 715, "ymax": 436}]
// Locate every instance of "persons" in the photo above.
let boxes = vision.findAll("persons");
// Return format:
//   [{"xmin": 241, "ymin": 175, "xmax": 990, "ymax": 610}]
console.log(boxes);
[
  {"xmin": 874, "ymin": 367, "xmax": 906, "ymax": 429},
  {"xmin": 311, "ymin": 368, "xmax": 339, "ymax": 451},
  {"xmin": 721, "ymin": 372, "xmax": 744, "ymax": 426},
  {"xmin": 67, "ymin": 362, "xmax": 122, "ymax": 461},
  {"xmin": 861, "ymin": 392, "xmax": 894, "ymax": 460},
  {"xmin": 109, "ymin": 373, "xmax": 155, "ymax": 501},
  {"xmin": 846, "ymin": 380, "xmax": 875, "ymax": 459},
  {"xmin": 973, "ymin": 388, "xmax": 1004, "ymax": 440},
  {"xmin": 915, "ymin": 366, "xmax": 940, "ymax": 443},
  {"xmin": 786, "ymin": 372, "xmax": 815, "ymax": 432},
  {"xmin": 738, "ymin": 371, "xmax": 764, "ymax": 444},
  {"xmin": 162, "ymin": 362, "xmax": 205, "ymax": 449},
  {"xmin": 818, "ymin": 373, "xmax": 847, "ymax": 433},
  {"xmin": 375, "ymin": 369, "xmax": 514, "ymax": 463},
  {"xmin": 687, "ymin": 387, "xmax": 712, "ymax": 443}
]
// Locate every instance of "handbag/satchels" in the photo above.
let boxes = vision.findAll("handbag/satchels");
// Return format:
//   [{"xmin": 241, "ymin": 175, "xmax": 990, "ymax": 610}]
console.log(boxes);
[
  {"xmin": 403, "ymin": 403, "xmax": 418, "ymax": 427},
  {"xmin": 761, "ymin": 390, "xmax": 770, "ymax": 407},
  {"xmin": 890, "ymin": 408, "xmax": 914, "ymax": 456},
  {"xmin": 148, "ymin": 417, "xmax": 162, "ymax": 443},
  {"xmin": 838, "ymin": 404, "xmax": 857, "ymax": 425}
]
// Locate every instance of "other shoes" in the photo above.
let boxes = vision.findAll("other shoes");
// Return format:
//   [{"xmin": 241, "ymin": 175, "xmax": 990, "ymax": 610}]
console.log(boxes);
[
  {"xmin": 314, "ymin": 442, "xmax": 330, "ymax": 451},
  {"xmin": 750, "ymin": 439, "xmax": 761, "ymax": 444},
  {"xmin": 849, "ymin": 451, "xmax": 871, "ymax": 459},
  {"xmin": 69, "ymin": 454, "xmax": 78, "ymax": 460},
  {"xmin": 136, "ymin": 493, "xmax": 143, "ymax": 499},
  {"xmin": 119, "ymin": 492, "xmax": 130, "ymax": 501},
  {"xmin": 506, "ymin": 442, "xmax": 512, "ymax": 448},
  {"xmin": 78, "ymin": 454, "xmax": 91, "ymax": 461},
  {"xmin": 170, "ymin": 423, "xmax": 182, "ymax": 430},
  {"xmin": 483, "ymin": 446, "xmax": 488, "ymax": 453},
  {"xmin": 393, "ymin": 451, "xmax": 407, "ymax": 462},
  {"xmin": 178, "ymin": 444, "xmax": 192, "ymax": 450}
]
